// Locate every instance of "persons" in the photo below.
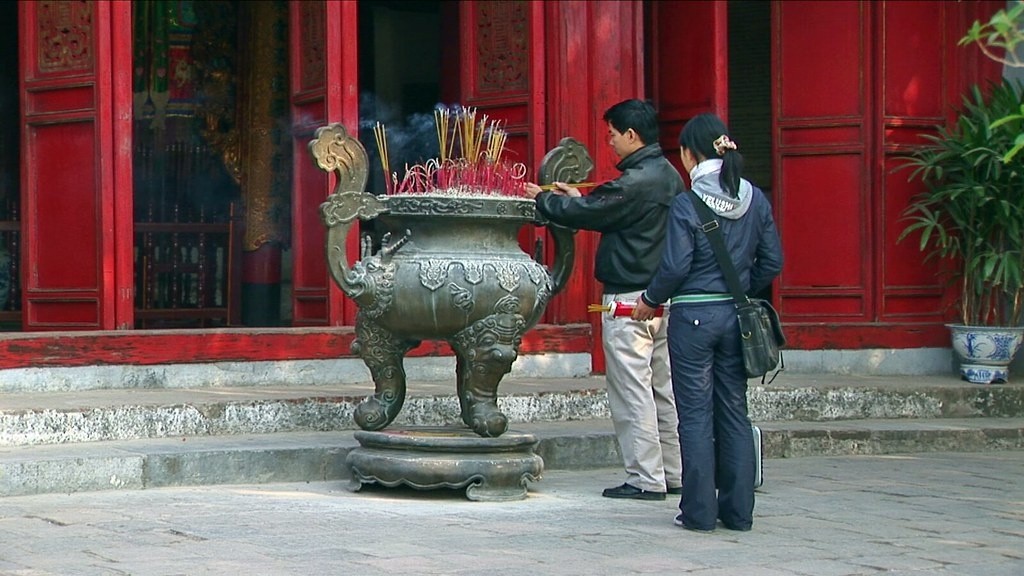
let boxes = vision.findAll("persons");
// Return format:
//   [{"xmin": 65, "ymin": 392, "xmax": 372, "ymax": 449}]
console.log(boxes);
[
  {"xmin": 631, "ymin": 112, "xmax": 783, "ymax": 533},
  {"xmin": 515, "ymin": 99, "xmax": 686, "ymax": 500}
]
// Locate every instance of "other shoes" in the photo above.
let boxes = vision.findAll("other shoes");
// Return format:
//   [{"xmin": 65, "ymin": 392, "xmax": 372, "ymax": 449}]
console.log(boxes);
[
  {"xmin": 718, "ymin": 518, "xmax": 751, "ymax": 531},
  {"xmin": 673, "ymin": 514, "xmax": 714, "ymax": 533}
]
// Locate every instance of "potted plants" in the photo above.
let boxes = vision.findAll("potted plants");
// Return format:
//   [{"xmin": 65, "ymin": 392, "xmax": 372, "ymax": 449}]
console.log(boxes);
[{"xmin": 889, "ymin": 76, "xmax": 1024, "ymax": 383}]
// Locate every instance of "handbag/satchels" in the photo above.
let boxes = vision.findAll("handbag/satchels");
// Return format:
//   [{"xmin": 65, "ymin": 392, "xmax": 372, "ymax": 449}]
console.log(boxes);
[{"xmin": 735, "ymin": 299, "xmax": 786, "ymax": 385}]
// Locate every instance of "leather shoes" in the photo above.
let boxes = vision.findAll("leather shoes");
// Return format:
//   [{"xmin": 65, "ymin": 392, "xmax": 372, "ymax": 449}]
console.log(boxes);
[
  {"xmin": 603, "ymin": 482, "xmax": 666, "ymax": 501},
  {"xmin": 666, "ymin": 487, "xmax": 682, "ymax": 495}
]
